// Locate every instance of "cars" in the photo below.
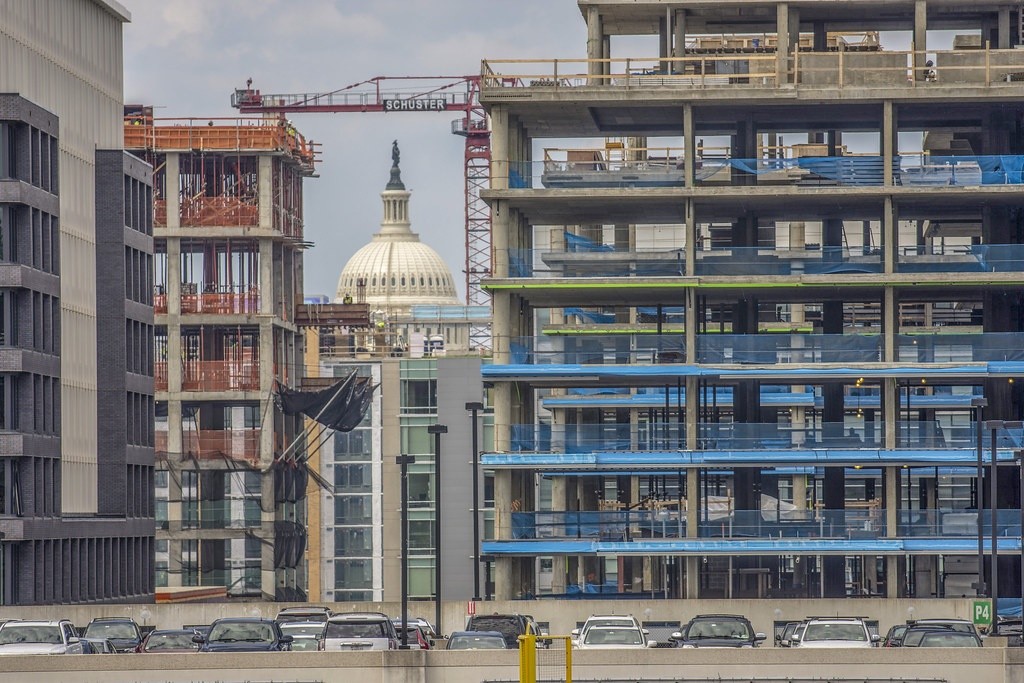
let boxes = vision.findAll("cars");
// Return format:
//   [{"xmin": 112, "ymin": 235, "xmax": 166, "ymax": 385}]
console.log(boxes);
[{"xmin": 0, "ymin": 608, "xmax": 983, "ymax": 655}]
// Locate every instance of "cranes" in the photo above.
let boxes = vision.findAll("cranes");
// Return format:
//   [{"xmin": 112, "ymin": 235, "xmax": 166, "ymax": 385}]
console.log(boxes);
[{"xmin": 230, "ymin": 78, "xmax": 523, "ymax": 360}]
